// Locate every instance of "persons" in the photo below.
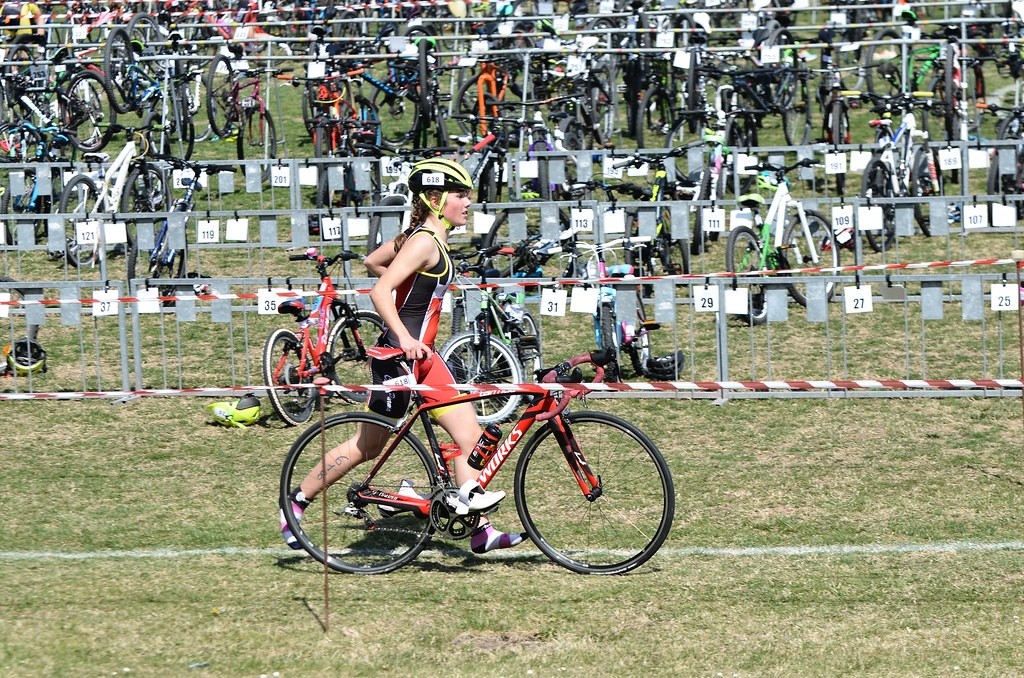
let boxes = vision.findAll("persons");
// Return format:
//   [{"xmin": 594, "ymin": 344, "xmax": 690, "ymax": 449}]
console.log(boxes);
[
  {"xmin": 279, "ymin": 158, "xmax": 530, "ymax": 554},
  {"xmin": 0, "ymin": 4, "xmax": 45, "ymax": 82}
]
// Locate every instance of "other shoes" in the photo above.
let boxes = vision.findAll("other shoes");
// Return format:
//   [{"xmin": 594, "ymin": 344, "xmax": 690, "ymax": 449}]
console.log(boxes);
[
  {"xmin": 377, "ymin": 480, "xmax": 432, "ymax": 513},
  {"xmin": 447, "ymin": 478, "xmax": 506, "ymax": 515}
]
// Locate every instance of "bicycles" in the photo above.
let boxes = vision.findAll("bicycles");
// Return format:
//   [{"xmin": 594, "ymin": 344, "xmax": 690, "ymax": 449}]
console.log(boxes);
[
  {"xmin": 279, "ymin": 346, "xmax": 675, "ymax": 576},
  {"xmin": 0, "ymin": 0, "xmax": 1024, "ymax": 426}
]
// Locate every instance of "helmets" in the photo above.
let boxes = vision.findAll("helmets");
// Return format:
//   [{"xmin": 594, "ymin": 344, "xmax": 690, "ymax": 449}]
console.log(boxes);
[
  {"xmin": 0, "ymin": 138, "xmax": 20, "ymax": 152},
  {"xmin": 206, "ymin": 392, "xmax": 261, "ymax": 428},
  {"xmin": 7, "ymin": 337, "xmax": 47, "ymax": 376},
  {"xmin": 408, "ymin": 158, "xmax": 474, "ymax": 194},
  {"xmin": 45, "ymin": 236, "xmax": 77, "ymax": 262},
  {"xmin": 193, "ymin": 284, "xmax": 208, "ymax": 294},
  {"xmin": 647, "ymin": 351, "xmax": 685, "ymax": 381}
]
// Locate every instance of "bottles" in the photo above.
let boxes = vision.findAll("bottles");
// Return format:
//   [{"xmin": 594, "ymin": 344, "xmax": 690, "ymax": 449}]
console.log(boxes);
[{"xmin": 468, "ymin": 424, "xmax": 502, "ymax": 471}]
